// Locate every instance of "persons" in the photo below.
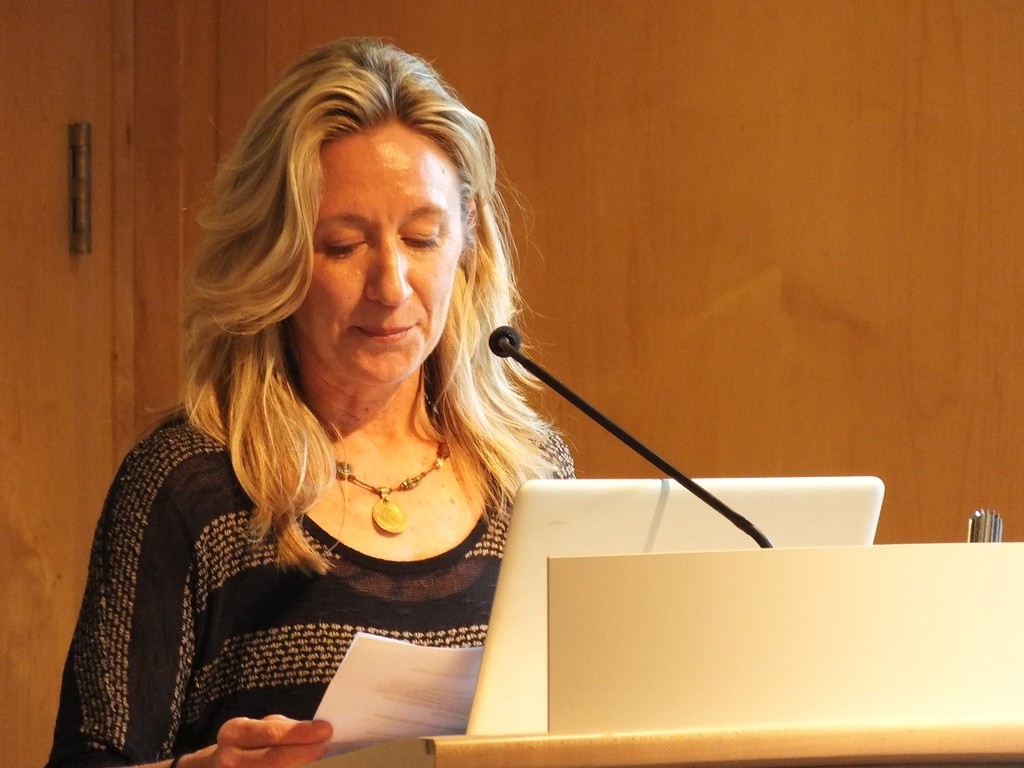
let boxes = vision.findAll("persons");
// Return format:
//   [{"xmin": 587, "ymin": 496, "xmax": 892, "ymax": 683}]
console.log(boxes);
[{"xmin": 42, "ymin": 34, "xmax": 576, "ymax": 768}]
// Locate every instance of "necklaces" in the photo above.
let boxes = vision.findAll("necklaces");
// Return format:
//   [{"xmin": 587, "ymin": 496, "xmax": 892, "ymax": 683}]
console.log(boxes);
[{"xmin": 332, "ymin": 390, "xmax": 451, "ymax": 535}]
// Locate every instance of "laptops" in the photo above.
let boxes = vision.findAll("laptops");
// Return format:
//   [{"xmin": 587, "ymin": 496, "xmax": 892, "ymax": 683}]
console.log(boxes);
[{"xmin": 463, "ymin": 473, "xmax": 884, "ymax": 739}]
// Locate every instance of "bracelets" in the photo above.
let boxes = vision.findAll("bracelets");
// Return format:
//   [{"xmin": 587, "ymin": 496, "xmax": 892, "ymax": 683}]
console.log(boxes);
[{"xmin": 168, "ymin": 751, "xmax": 185, "ymax": 768}]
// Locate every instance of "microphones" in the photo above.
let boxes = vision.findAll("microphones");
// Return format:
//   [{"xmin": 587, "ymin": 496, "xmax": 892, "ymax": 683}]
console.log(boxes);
[{"xmin": 489, "ymin": 326, "xmax": 776, "ymax": 545}]
